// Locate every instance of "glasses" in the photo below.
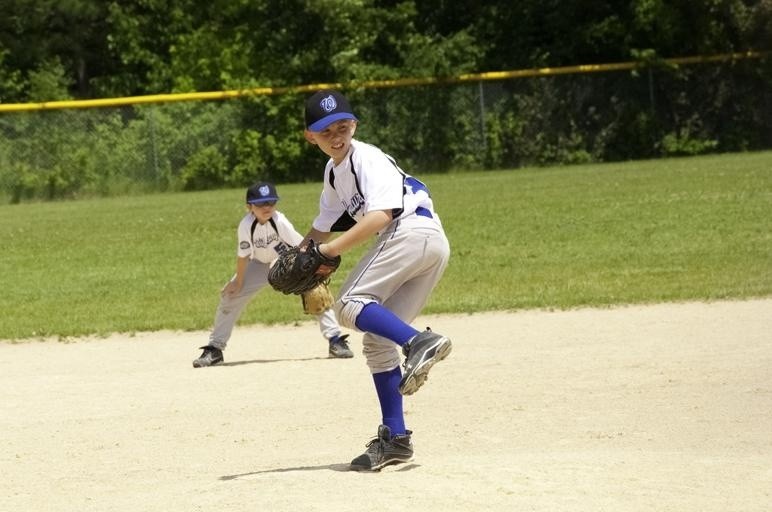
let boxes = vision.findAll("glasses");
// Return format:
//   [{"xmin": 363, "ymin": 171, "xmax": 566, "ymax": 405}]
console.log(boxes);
[{"xmin": 252, "ymin": 201, "xmax": 277, "ymax": 208}]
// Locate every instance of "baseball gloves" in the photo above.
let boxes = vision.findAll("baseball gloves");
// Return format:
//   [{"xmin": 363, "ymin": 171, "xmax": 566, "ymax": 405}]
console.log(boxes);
[
  {"xmin": 268, "ymin": 238, "xmax": 341, "ymax": 295},
  {"xmin": 302, "ymin": 278, "xmax": 335, "ymax": 314}
]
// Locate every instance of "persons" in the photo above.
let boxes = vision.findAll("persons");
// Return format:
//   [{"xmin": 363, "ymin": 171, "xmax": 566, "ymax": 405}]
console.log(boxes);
[
  {"xmin": 192, "ymin": 182, "xmax": 354, "ymax": 367},
  {"xmin": 267, "ymin": 90, "xmax": 453, "ymax": 471}
]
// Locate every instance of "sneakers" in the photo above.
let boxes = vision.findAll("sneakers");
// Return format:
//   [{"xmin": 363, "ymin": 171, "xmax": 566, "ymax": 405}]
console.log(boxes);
[
  {"xmin": 397, "ymin": 331, "xmax": 451, "ymax": 396},
  {"xmin": 327, "ymin": 335, "xmax": 352, "ymax": 357},
  {"xmin": 193, "ymin": 345, "xmax": 222, "ymax": 367},
  {"xmin": 350, "ymin": 425, "xmax": 414, "ymax": 469}
]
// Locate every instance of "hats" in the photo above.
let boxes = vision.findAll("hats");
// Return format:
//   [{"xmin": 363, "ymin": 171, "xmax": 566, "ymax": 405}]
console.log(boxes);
[
  {"xmin": 246, "ymin": 182, "xmax": 279, "ymax": 203},
  {"xmin": 304, "ymin": 90, "xmax": 358, "ymax": 132}
]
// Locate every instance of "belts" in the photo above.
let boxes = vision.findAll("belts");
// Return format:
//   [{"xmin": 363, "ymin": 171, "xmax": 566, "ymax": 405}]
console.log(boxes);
[{"xmin": 415, "ymin": 205, "xmax": 434, "ymax": 219}]
